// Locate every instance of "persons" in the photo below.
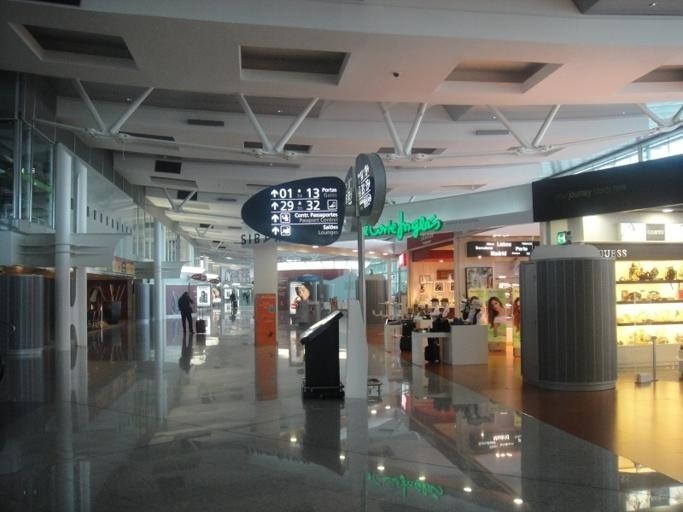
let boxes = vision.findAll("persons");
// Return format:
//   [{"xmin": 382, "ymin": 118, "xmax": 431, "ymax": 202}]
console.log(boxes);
[
  {"xmin": 440, "ymin": 297, "xmax": 452, "ymax": 319},
  {"xmin": 230, "ymin": 289, "xmax": 235, "ymax": 306},
  {"xmin": 178, "ymin": 292, "xmax": 194, "ymax": 333},
  {"xmin": 178, "ymin": 332, "xmax": 194, "ymax": 373},
  {"xmin": 293, "ymin": 279, "xmax": 311, "ymax": 300},
  {"xmin": 430, "ymin": 297, "xmax": 439, "ymax": 312},
  {"xmin": 198, "ymin": 291, "xmax": 207, "ymax": 303},
  {"xmin": 488, "ymin": 297, "xmax": 507, "ymax": 335},
  {"xmin": 512, "ymin": 297, "xmax": 521, "ymax": 332}
]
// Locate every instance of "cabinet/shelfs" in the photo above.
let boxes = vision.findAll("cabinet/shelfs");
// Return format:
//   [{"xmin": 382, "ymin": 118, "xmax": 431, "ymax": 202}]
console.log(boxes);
[
  {"xmin": 381, "ymin": 318, "xmax": 403, "ymax": 353},
  {"xmin": 616, "ymin": 279, "xmax": 683, "ymax": 326},
  {"xmin": 410, "ymin": 323, "xmax": 489, "ymax": 367}
]
